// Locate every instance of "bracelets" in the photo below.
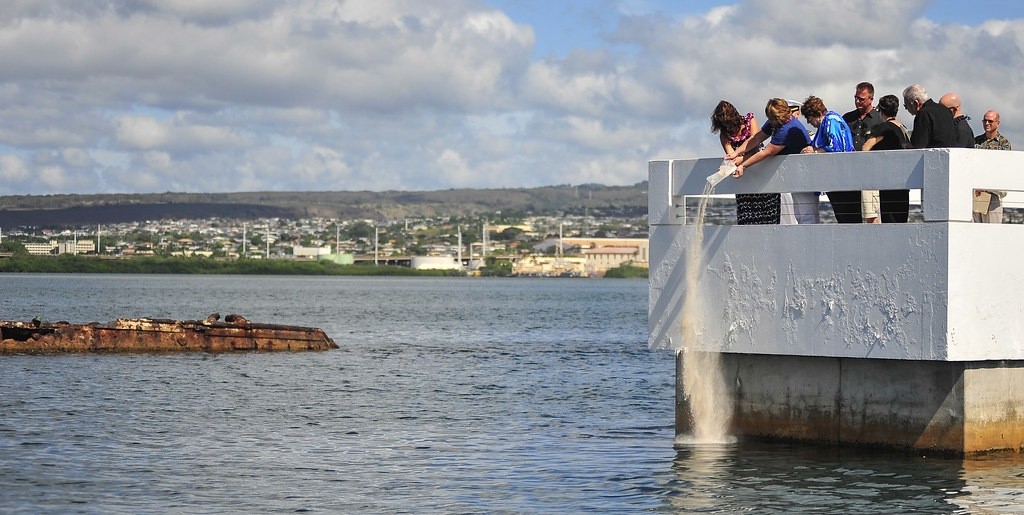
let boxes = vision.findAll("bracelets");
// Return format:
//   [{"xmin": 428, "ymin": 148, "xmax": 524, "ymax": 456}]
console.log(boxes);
[{"xmin": 814, "ymin": 149, "xmax": 818, "ymax": 153}]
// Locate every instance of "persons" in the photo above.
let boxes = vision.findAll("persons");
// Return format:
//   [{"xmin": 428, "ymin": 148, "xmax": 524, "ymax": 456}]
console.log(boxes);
[
  {"xmin": 901, "ymin": 84, "xmax": 956, "ymax": 149},
  {"xmin": 842, "ymin": 81, "xmax": 885, "ymax": 223},
  {"xmin": 800, "ymin": 96, "xmax": 862, "ymax": 223},
  {"xmin": 973, "ymin": 110, "xmax": 1011, "ymax": 224},
  {"xmin": 725, "ymin": 98, "xmax": 820, "ymax": 224},
  {"xmin": 861, "ymin": 95, "xmax": 910, "ymax": 223},
  {"xmin": 712, "ymin": 100, "xmax": 781, "ymax": 225},
  {"xmin": 940, "ymin": 93, "xmax": 975, "ymax": 149},
  {"xmin": 760, "ymin": 100, "xmax": 816, "ymax": 224}
]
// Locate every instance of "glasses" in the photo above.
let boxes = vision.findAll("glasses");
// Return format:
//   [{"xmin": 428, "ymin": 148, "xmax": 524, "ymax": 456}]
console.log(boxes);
[
  {"xmin": 982, "ymin": 120, "xmax": 998, "ymax": 123},
  {"xmin": 854, "ymin": 96, "xmax": 865, "ymax": 101},
  {"xmin": 903, "ymin": 100, "xmax": 915, "ymax": 108}
]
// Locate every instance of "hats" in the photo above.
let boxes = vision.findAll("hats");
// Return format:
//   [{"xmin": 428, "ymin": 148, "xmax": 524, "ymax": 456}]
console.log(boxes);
[{"xmin": 786, "ymin": 100, "xmax": 804, "ymax": 114}]
